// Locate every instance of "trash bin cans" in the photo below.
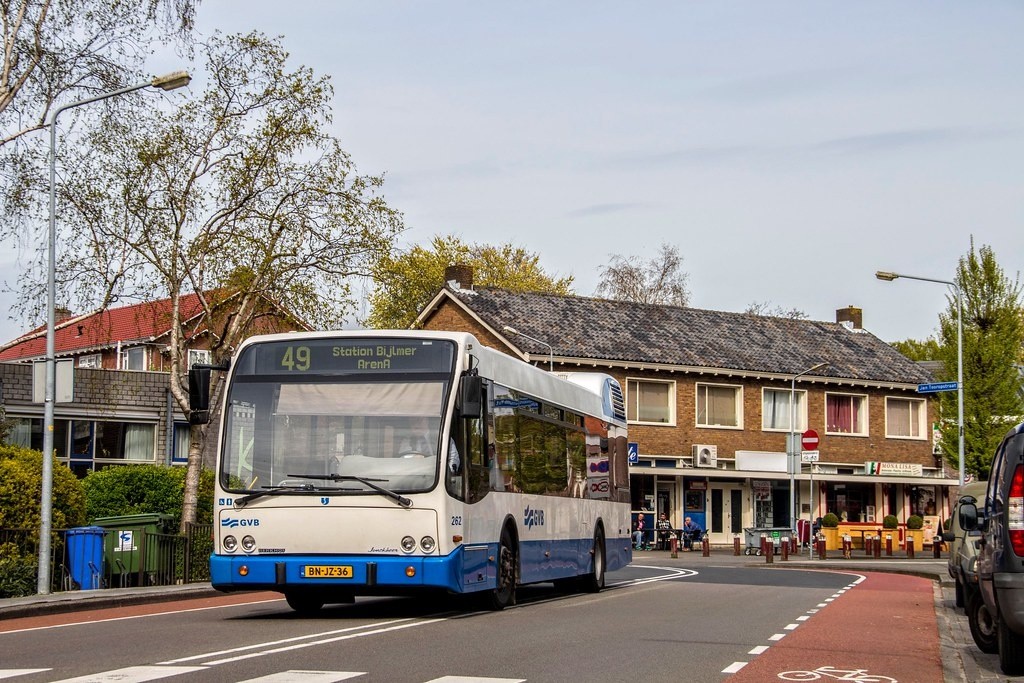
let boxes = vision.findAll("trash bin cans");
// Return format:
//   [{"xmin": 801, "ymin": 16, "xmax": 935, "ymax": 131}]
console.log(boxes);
[
  {"xmin": 66, "ymin": 527, "xmax": 105, "ymax": 589},
  {"xmin": 744, "ymin": 528, "xmax": 793, "ymax": 556},
  {"xmin": 91, "ymin": 513, "xmax": 178, "ymax": 586}
]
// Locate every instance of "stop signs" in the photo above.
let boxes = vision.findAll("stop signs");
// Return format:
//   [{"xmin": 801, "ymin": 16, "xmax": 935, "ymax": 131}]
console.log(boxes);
[{"xmin": 801, "ymin": 429, "xmax": 820, "ymax": 451}]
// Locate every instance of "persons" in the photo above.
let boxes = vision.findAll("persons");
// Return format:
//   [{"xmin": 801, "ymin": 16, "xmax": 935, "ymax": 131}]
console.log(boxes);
[
  {"xmin": 632, "ymin": 513, "xmax": 652, "ymax": 551},
  {"xmin": 682, "ymin": 517, "xmax": 703, "ymax": 551},
  {"xmin": 656, "ymin": 513, "xmax": 672, "ymax": 546},
  {"xmin": 398, "ymin": 411, "xmax": 460, "ymax": 476}
]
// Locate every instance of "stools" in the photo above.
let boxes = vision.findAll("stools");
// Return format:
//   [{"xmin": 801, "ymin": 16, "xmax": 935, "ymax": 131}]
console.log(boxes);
[{"xmin": 683, "ymin": 530, "xmax": 701, "ymax": 550}]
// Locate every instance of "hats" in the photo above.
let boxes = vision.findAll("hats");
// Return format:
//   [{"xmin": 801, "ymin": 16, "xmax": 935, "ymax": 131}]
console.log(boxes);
[{"xmin": 661, "ymin": 512, "xmax": 666, "ymax": 515}]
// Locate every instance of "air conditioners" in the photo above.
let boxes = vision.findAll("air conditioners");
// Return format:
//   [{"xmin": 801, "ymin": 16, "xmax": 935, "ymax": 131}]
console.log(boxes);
[{"xmin": 693, "ymin": 445, "xmax": 717, "ymax": 468}]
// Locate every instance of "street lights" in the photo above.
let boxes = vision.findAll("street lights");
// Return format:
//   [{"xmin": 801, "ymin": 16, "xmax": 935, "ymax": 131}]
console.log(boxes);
[
  {"xmin": 876, "ymin": 271, "xmax": 964, "ymax": 487},
  {"xmin": 790, "ymin": 363, "xmax": 828, "ymax": 529},
  {"xmin": 504, "ymin": 326, "xmax": 553, "ymax": 371},
  {"xmin": 36, "ymin": 71, "xmax": 192, "ymax": 593}
]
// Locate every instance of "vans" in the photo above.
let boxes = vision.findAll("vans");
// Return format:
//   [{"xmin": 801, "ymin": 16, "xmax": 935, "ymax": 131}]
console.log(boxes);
[{"xmin": 958, "ymin": 422, "xmax": 1024, "ymax": 677}]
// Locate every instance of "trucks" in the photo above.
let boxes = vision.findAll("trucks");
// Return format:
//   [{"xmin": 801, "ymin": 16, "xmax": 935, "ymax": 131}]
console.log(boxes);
[{"xmin": 943, "ymin": 482, "xmax": 989, "ymax": 617}]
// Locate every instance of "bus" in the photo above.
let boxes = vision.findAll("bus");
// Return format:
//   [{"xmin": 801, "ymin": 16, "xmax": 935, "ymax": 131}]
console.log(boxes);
[{"xmin": 188, "ymin": 330, "xmax": 632, "ymax": 614}]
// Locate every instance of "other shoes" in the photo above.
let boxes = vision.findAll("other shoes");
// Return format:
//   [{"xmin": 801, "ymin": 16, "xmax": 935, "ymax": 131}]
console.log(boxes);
[
  {"xmin": 636, "ymin": 546, "xmax": 642, "ymax": 551},
  {"xmin": 646, "ymin": 546, "xmax": 651, "ymax": 550},
  {"xmin": 682, "ymin": 547, "xmax": 690, "ymax": 551}
]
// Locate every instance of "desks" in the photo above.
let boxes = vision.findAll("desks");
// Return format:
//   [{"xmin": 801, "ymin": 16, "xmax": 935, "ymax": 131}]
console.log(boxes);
[
  {"xmin": 851, "ymin": 529, "xmax": 893, "ymax": 550},
  {"xmin": 643, "ymin": 528, "xmax": 683, "ymax": 551}
]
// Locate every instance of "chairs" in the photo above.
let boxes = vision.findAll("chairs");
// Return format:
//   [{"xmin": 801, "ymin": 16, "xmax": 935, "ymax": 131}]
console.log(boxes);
[{"xmin": 689, "ymin": 529, "xmax": 709, "ymax": 551}]
